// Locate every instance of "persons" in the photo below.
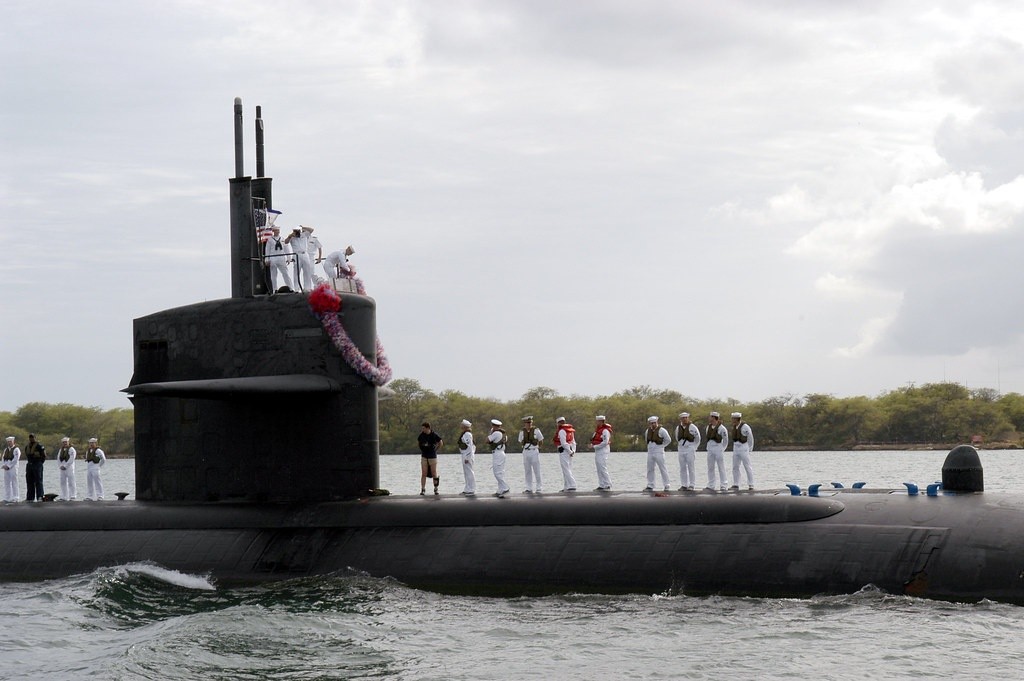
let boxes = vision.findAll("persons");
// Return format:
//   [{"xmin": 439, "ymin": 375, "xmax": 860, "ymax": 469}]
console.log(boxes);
[
  {"xmin": 265, "ymin": 225, "xmax": 298, "ymax": 294},
  {"xmin": 553, "ymin": 417, "xmax": 576, "ymax": 493},
  {"xmin": 23, "ymin": 433, "xmax": 47, "ymax": 503},
  {"xmin": 417, "ymin": 422, "xmax": 443, "ymax": 495},
  {"xmin": 84, "ymin": 438, "xmax": 107, "ymax": 501},
  {"xmin": 485, "ymin": 419, "xmax": 510, "ymax": 498},
  {"xmin": 53, "ymin": 437, "xmax": 77, "ymax": 501},
  {"xmin": 643, "ymin": 416, "xmax": 671, "ymax": 492},
  {"xmin": 457, "ymin": 419, "xmax": 476, "ymax": 496},
  {"xmin": 285, "ymin": 225, "xmax": 322, "ymax": 293},
  {"xmin": 675, "ymin": 412, "xmax": 701, "ymax": 491},
  {"xmin": 517, "ymin": 416, "xmax": 544, "ymax": 494},
  {"xmin": 728, "ymin": 412, "xmax": 754, "ymax": 491},
  {"xmin": 588, "ymin": 415, "xmax": 613, "ymax": 491},
  {"xmin": 323, "ymin": 246, "xmax": 355, "ymax": 279},
  {"xmin": 1, "ymin": 437, "xmax": 21, "ymax": 503},
  {"xmin": 703, "ymin": 412, "xmax": 728, "ymax": 492}
]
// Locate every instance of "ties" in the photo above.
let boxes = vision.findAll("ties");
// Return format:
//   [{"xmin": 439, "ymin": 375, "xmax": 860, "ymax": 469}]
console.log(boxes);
[{"xmin": 272, "ymin": 236, "xmax": 282, "ymax": 250}]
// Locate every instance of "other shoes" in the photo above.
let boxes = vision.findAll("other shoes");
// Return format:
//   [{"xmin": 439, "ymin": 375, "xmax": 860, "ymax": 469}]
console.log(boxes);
[
  {"xmin": 641, "ymin": 487, "xmax": 755, "ymax": 490},
  {"xmin": 2, "ymin": 496, "xmax": 103, "ymax": 501},
  {"xmin": 419, "ymin": 488, "xmax": 611, "ymax": 499}
]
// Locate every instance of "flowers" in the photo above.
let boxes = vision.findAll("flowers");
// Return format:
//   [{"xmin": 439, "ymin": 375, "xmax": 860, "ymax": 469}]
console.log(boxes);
[{"xmin": 307, "ymin": 276, "xmax": 392, "ymax": 387}]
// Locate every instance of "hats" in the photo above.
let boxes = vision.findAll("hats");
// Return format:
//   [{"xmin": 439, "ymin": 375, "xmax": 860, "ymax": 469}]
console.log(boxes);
[
  {"xmin": 648, "ymin": 412, "xmax": 744, "ymax": 424},
  {"xmin": 5, "ymin": 433, "xmax": 101, "ymax": 442},
  {"xmin": 461, "ymin": 416, "xmax": 608, "ymax": 425},
  {"xmin": 272, "ymin": 225, "xmax": 280, "ymax": 229},
  {"xmin": 293, "ymin": 228, "xmax": 300, "ymax": 231},
  {"xmin": 350, "ymin": 244, "xmax": 356, "ymax": 254}
]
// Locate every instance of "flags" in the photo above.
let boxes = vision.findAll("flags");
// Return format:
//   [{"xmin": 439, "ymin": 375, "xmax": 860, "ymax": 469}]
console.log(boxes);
[{"xmin": 254, "ymin": 208, "xmax": 274, "ymax": 245}]
